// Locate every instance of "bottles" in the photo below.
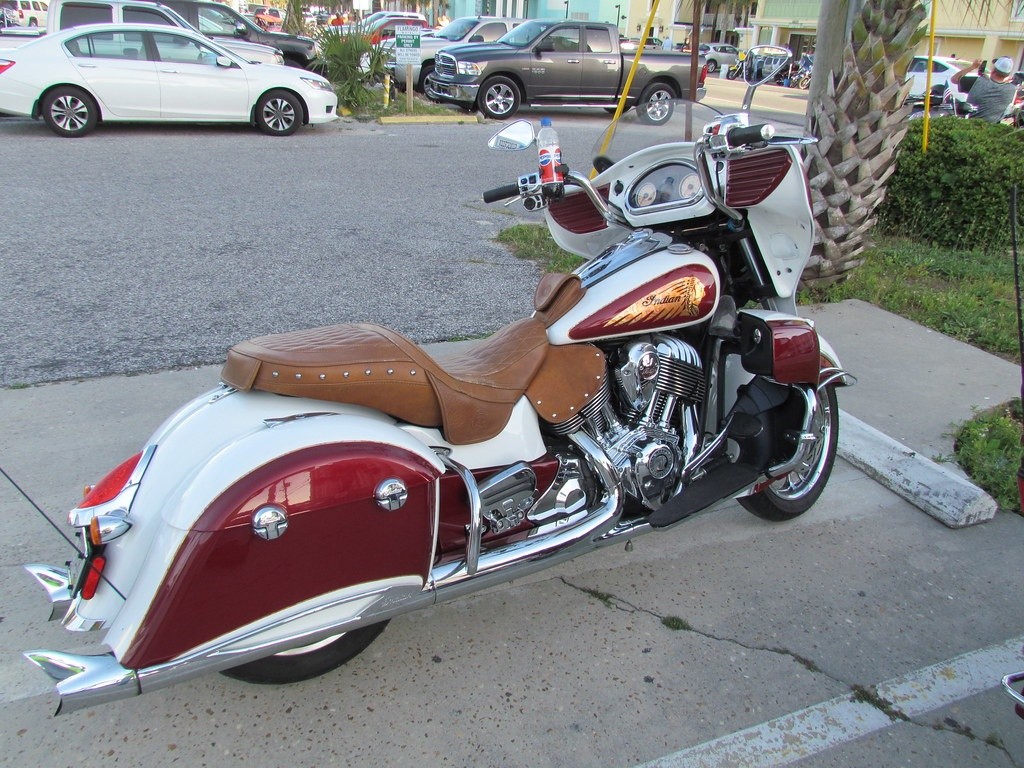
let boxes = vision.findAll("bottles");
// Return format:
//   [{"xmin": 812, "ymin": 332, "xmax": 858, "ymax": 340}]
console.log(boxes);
[{"xmin": 537, "ymin": 118, "xmax": 565, "ymax": 199}]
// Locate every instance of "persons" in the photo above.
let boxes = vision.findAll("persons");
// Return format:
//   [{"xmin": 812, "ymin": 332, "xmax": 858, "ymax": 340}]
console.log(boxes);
[
  {"xmin": 757, "ymin": 57, "xmax": 762, "ymax": 80},
  {"xmin": 746, "ymin": 54, "xmax": 753, "ymax": 78},
  {"xmin": 785, "ymin": 43, "xmax": 794, "ymax": 54},
  {"xmin": 682, "ymin": 37, "xmax": 692, "ymax": 53},
  {"xmin": 720, "ymin": 47, "xmax": 726, "ymax": 52},
  {"xmin": 661, "ymin": 37, "xmax": 674, "ymax": 50},
  {"xmin": 802, "ymin": 47, "xmax": 815, "ymax": 54},
  {"xmin": 951, "ymin": 56, "xmax": 1024, "ymax": 131},
  {"xmin": 331, "ymin": 13, "xmax": 344, "ymax": 27}
]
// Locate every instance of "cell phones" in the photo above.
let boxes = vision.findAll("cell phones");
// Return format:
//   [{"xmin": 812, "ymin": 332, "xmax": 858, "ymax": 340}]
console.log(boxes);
[{"xmin": 979, "ymin": 60, "xmax": 987, "ymax": 73}]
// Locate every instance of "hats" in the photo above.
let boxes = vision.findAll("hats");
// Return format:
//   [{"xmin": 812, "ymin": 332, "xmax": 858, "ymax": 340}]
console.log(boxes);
[{"xmin": 992, "ymin": 56, "xmax": 1013, "ymax": 77}]
